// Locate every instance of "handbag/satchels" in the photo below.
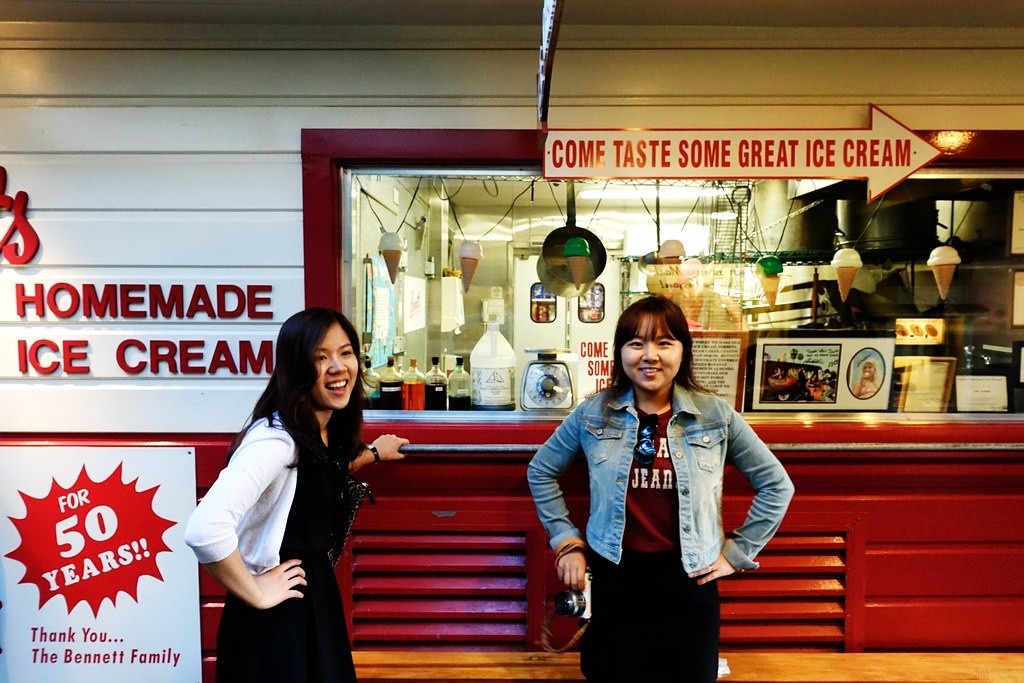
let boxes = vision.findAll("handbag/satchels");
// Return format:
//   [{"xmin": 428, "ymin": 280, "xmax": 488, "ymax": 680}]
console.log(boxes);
[{"xmin": 326, "ymin": 472, "xmax": 375, "ymax": 571}]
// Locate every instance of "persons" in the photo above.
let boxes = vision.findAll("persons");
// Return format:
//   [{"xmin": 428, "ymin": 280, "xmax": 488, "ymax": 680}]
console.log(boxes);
[
  {"xmin": 185, "ymin": 307, "xmax": 408, "ymax": 683},
  {"xmin": 527, "ymin": 297, "xmax": 795, "ymax": 683},
  {"xmin": 853, "ymin": 357, "xmax": 882, "ymax": 398}
]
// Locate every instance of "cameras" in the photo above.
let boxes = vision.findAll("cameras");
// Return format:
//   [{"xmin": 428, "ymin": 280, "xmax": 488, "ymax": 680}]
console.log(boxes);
[{"xmin": 555, "ymin": 572, "xmax": 593, "ymax": 619}]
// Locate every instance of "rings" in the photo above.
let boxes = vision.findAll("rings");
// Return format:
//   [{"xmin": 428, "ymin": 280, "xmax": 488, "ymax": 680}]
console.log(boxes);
[{"xmin": 710, "ymin": 565, "xmax": 713, "ymax": 571}]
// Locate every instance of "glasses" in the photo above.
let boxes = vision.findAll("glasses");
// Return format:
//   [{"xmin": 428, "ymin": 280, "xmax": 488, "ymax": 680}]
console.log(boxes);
[
  {"xmin": 633, "ymin": 414, "xmax": 658, "ymax": 468},
  {"xmin": 327, "ymin": 456, "xmax": 353, "ymax": 514}
]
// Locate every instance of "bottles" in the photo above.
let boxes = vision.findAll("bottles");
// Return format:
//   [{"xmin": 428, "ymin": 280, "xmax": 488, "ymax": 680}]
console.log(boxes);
[
  {"xmin": 363, "ymin": 342, "xmax": 372, "ymax": 360},
  {"xmin": 360, "ymin": 355, "xmax": 382, "ymax": 410},
  {"xmin": 423, "ymin": 356, "xmax": 448, "ymax": 411},
  {"xmin": 447, "ymin": 357, "xmax": 471, "ymax": 411},
  {"xmin": 402, "ymin": 358, "xmax": 425, "ymax": 411},
  {"xmin": 379, "ymin": 355, "xmax": 405, "ymax": 410},
  {"xmin": 468, "ymin": 322, "xmax": 518, "ymax": 411}
]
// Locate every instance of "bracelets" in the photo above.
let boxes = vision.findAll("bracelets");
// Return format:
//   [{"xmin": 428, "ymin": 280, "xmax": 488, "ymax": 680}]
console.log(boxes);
[{"xmin": 366, "ymin": 444, "xmax": 380, "ymax": 464}]
